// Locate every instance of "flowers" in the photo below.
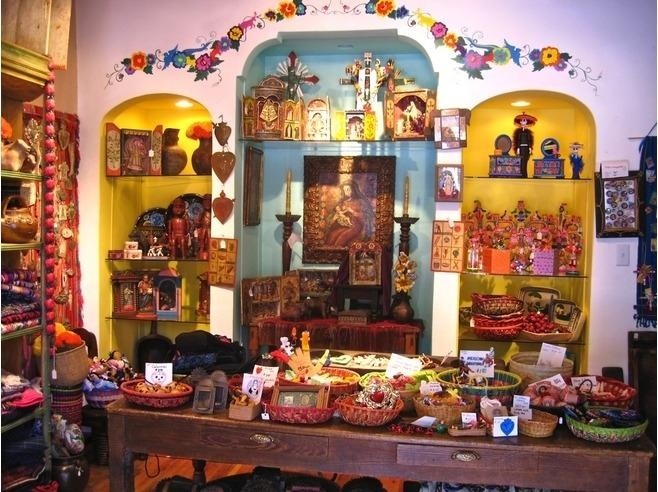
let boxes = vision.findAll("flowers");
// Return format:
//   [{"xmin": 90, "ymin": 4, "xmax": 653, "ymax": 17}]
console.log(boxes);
[
  {"xmin": 394, "ymin": 251, "xmax": 417, "ymax": 294},
  {"xmin": 186, "ymin": 117, "xmax": 212, "ymax": 139}
]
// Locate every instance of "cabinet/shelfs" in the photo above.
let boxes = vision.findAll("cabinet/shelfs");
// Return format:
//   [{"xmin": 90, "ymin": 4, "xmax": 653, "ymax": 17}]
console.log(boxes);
[
  {"xmin": 247, "ymin": 318, "xmax": 423, "ymax": 356},
  {"xmin": 233, "ymin": 32, "xmax": 439, "ymax": 356},
  {"xmin": 456, "ymin": 89, "xmax": 596, "ymax": 375},
  {"xmin": 100, "ymin": 94, "xmax": 212, "ymax": 362},
  {"xmin": 2, "ymin": 41, "xmax": 54, "ymax": 491}
]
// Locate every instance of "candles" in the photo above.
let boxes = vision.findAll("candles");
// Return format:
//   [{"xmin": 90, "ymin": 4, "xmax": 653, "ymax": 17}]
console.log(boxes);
[
  {"xmin": 404, "ymin": 176, "xmax": 409, "ymax": 216},
  {"xmin": 286, "ymin": 169, "xmax": 292, "ymax": 213}
]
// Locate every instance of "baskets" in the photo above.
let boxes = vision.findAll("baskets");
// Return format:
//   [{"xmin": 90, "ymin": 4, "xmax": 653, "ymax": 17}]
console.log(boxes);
[
  {"xmin": 262, "ymin": 352, "xmax": 576, "ymax": 436},
  {"xmin": 471, "ymin": 291, "xmax": 525, "ymax": 339},
  {"xmin": 36, "ymin": 340, "xmax": 89, "ymax": 387},
  {"xmin": 262, "ymin": 352, "xmax": 648, "ymax": 442}
]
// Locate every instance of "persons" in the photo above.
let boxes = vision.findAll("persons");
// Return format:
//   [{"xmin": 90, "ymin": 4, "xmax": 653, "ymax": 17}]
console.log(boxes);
[
  {"xmin": 324, "ymin": 179, "xmax": 373, "ymax": 247},
  {"xmin": 438, "ymin": 170, "xmax": 460, "ymax": 198},
  {"xmin": 569, "ymin": 141, "xmax": 584, "ymax": 179},
  {"xmin": 512, "ymin": 112, "xmax": 538, "ymax": 178},
  {"xmin": 249, "ymin": 380, "xmax": 258, "ymax": 395},
  {"xmin": 167, "ymin": 197, "xmax": 190, "ymax": 258}
]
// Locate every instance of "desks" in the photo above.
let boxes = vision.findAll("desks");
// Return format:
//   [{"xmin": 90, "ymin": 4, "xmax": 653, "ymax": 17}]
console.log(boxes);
[{"xmin": 105, "ymin": 399, "xmax": 653, "ymax": 492}]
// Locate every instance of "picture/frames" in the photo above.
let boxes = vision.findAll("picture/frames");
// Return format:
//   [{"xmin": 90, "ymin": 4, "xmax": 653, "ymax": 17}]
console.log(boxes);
[
  {"xmin": 211, "ymin": 370, "xmax": 228, "ymax": 411},
  {"xmin": 434, "ymin": 165, "xmax": 464, "ymax": 202},
  {"xmin": 243, "ymin": 145, "xmax": 264, "ymax": 225},
  {"xmin": 193, "ymin": 380, "xmax": 216, "ymax": 415},
  {"xmin": 303, "ymin": 156, "xmax": 395, "ymax": 264},
  {"xmin": 271, "ymin": 385, "xmax": 330, "ymax": 409},
  {"xmin": 594, "ymin": 170, "xmax": 645, "ymax": 238},
  {"xmin": 348, "ymin": 240, "xmax": 382, "ymax": 286}
]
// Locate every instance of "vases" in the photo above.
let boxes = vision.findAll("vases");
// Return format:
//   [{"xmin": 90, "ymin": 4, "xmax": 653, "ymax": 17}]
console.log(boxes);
[
  {"xmin": 192, "ymin": 139, "xmax": 212, "ymax": 175},
  {"xmin": 392, "ymin": 297, "xmax": 414, "ymax": 323}
]
